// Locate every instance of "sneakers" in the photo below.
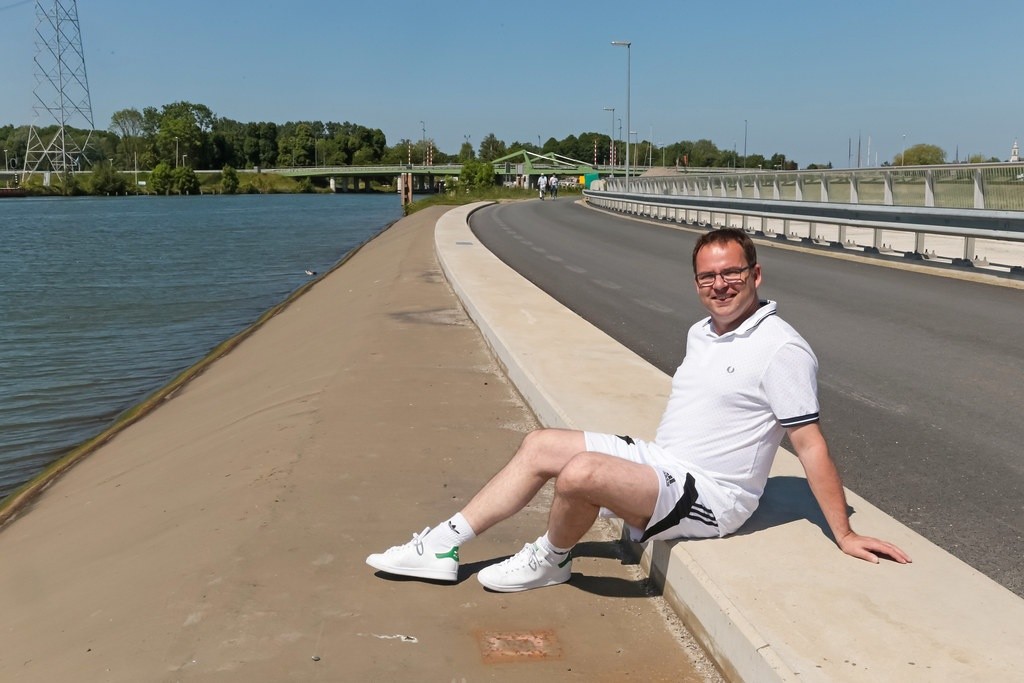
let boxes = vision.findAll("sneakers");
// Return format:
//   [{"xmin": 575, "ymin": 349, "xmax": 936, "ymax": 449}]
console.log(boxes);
[
  {"xmin": 365, "ymin": 527, "xmax": 458, "ymax": 581},
  {"xmin": 477, "ymin": 537, "xmax": 573, "ymax": 592}
]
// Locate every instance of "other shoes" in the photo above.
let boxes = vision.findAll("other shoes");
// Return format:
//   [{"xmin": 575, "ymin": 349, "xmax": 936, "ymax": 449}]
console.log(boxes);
[
  {"xmin": 539, "ymin": 197, "xmax": 542, "ymax": 199},
  {"xmin": 544, "ymin": 191, "xmax": 547, "ymax": 195}
]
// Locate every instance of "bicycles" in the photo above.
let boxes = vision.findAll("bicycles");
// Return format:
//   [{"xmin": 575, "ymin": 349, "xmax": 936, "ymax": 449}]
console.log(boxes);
[{"xmin": 538, "ymin": 179, "xmax": 558, "ymax": 201}]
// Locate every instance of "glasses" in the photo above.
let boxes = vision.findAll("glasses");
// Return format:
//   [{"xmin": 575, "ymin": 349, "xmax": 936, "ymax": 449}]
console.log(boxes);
[{"xmin": 695, "ymin": 264, "xmax": 753, "ymax": 288}]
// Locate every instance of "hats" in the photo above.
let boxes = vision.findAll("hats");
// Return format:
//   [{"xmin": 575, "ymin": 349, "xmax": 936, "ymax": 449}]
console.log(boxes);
[{"xmin": 541, "ymin": 173, "xmax": 544, "ymax": 175}]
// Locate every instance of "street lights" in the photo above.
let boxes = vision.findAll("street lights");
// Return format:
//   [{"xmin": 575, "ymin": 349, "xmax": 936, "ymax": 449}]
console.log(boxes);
[
  {"xmin": 629, "ymin": 131, "xmax": 638, "ymax": 179},
  {"xmin": 538, "ymin": 134, "xmax": 541, "ymax": 156},
  {"xmin": 183, "ymin": 154, "xmax": 188, "ymax": 168},
  {"xmin": 175, "ymin": 137, "xmax": 179, "ymax": 168},
  {"xmin": 901, "ymin": 134, "xmax": 906, "ymax": 168},
  {"xmin": 4, "ymin": 149, "xmax": 8, "ymax": 172},
  {"xmin": 611, "ymin": 40, "xmax": 631, "ymax": 193},
  {"xmin": 463, "ymin": 134, "xmax": 471, "ymax": 161},
  {"xmin": 603, "ymin": 107, "xmax": 615, "ymax": 178},
  {"xmin": 743, "ymin": 119, "xmax": 748, "ymax": 170},
  {"xmin": 420, "ymin": 120, "xmax": 426, "ymax": 168}
]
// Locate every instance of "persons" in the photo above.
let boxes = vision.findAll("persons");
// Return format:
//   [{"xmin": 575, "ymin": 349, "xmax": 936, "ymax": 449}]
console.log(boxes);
[
  {"xmin": 549, "ymin": 173, "xmax": 558, "ymax": 198},
  {"xmin": 537, "ymin": 173, "xmax": 547, "ymax": 199},
  {"xmin": 363, "ymin": 227, "xmax": 914, "ymax": 593}
]
